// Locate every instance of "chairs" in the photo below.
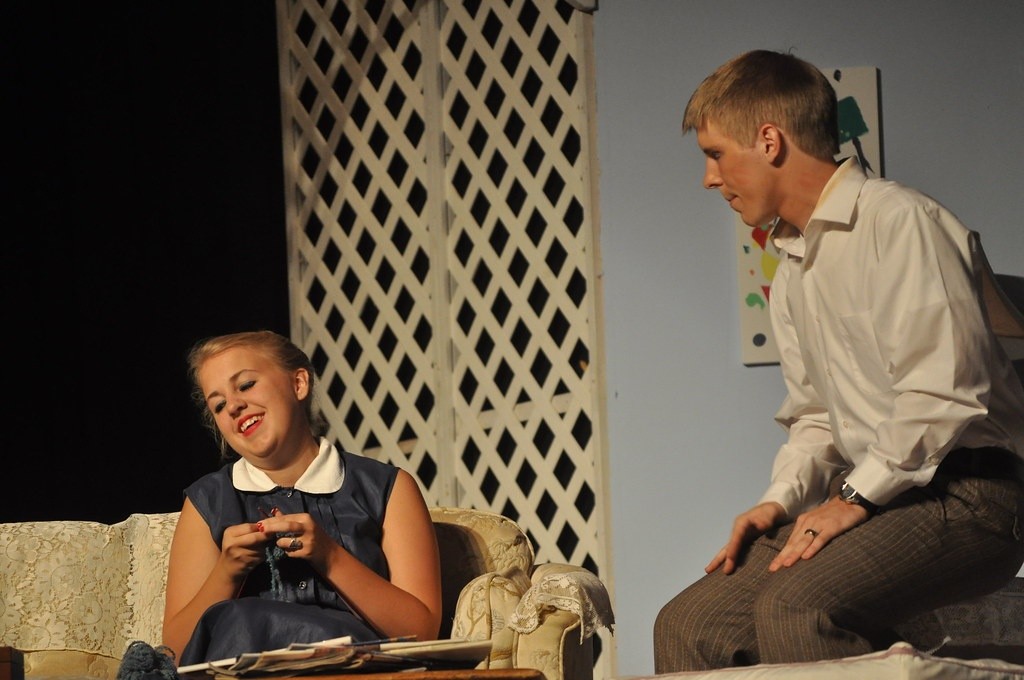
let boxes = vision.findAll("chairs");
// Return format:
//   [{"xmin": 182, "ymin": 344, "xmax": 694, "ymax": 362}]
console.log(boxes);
[{"xmin": 885, "ymin": 275, "xmax": 1024, "ymax": 665}]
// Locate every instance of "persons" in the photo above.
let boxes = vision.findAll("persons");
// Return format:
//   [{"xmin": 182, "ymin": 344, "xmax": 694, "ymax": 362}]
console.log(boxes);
[
  {"xmin": 161, "ymin": 333, "xmax": 442, "ymax": 666},
  {"xmin": 653, "ymin": 50, "xmax": 1024, "ymax": 676}
]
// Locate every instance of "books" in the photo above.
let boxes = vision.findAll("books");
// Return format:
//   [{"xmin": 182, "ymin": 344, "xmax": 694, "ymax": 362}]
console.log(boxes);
[{"xmin": 174, "ymin": 634, "xmax": 493, "ymax": 678}]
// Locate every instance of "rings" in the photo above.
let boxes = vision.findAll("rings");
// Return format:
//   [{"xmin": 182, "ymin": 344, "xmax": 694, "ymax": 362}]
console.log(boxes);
[
  {"xmin": 805, "ymin": 528, "xmax": 817, "ymax": 537},
  {"xmin": 288, "ymin": 539, "xmax": 303, "ymax": 550}
]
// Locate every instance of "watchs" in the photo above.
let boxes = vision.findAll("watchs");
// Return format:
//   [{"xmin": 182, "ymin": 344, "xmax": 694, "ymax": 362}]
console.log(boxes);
[{"xmin": 839, "ymin": 478, "xmax": 876, "ymax": 514}]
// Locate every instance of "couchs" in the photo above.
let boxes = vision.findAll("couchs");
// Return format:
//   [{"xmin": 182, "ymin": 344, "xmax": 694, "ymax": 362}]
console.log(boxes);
[{"xmin": 0, "ymin": 508, "xmax": 617, "ymax": 680}]
